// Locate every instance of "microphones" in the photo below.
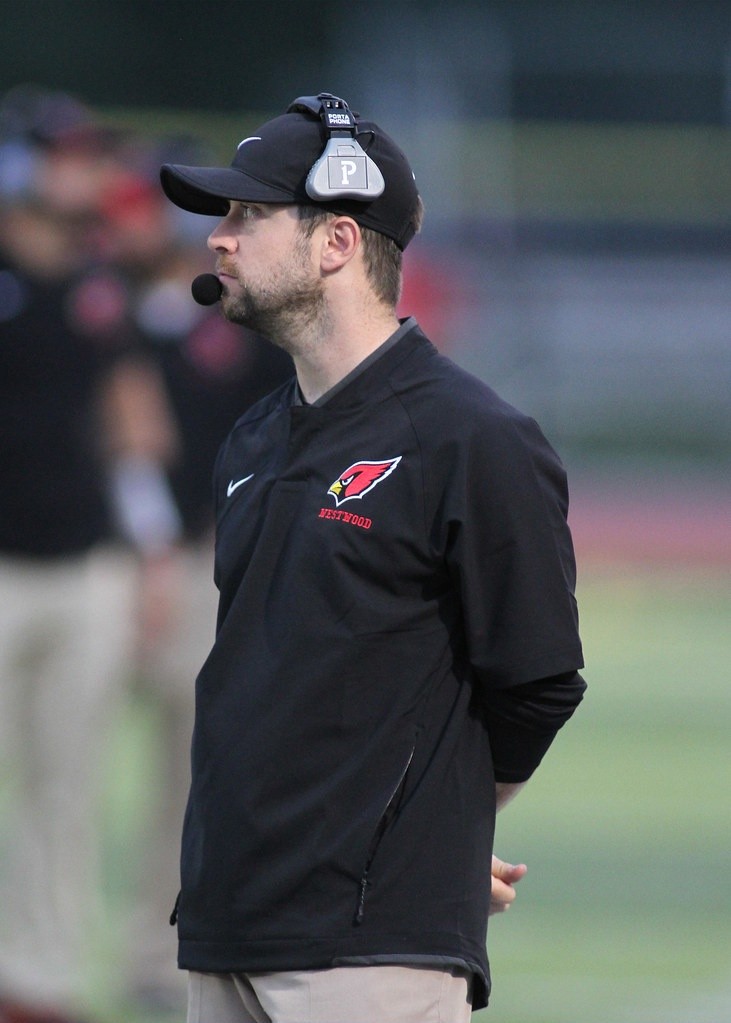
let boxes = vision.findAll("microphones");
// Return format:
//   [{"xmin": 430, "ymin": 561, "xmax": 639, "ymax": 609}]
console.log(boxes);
[{"xmin": 190, "ymin": 272, "xmax": 222, "ymax": 305}]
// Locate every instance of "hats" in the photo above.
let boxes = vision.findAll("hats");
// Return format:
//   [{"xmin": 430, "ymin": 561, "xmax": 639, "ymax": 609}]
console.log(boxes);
[{"xmin": 159, "ymin": 113, "xmax": 420, "ymax": 252}]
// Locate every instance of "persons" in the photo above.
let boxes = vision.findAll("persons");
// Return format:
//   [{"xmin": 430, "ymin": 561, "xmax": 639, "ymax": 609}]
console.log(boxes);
[
  {"xmin": 153, "ymin": 89, "xmax": 586, "ymax": 1022},
  {"xmin": 0, "ymin": 141, "xmax": 281, "ymax": 1023}
]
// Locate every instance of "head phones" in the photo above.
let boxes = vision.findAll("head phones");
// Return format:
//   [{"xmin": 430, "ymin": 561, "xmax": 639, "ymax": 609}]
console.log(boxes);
[{"xmin": 285, "ymin": 93, "xmax": 385, "ymax": 202}]
